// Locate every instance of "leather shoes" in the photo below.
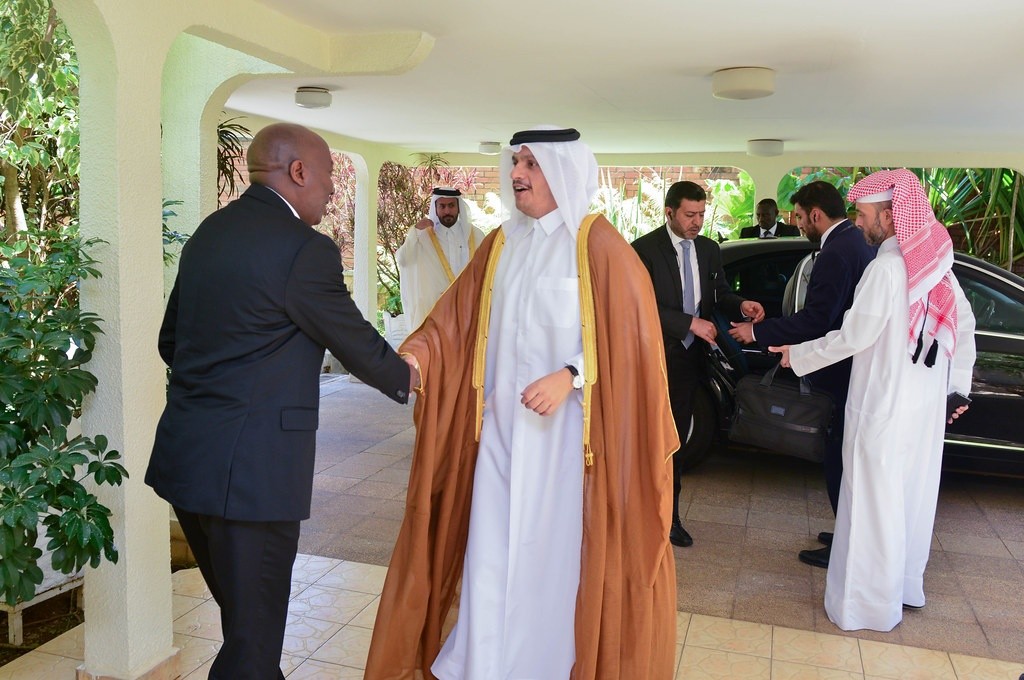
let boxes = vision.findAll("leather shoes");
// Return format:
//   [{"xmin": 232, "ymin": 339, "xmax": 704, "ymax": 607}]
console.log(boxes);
[
  {"xmin": 818, "ymin": 532, "xmax": 835, "ymax": 545},
  {"xmin": 669, "ymin": 520, "xmax": 692, "ymax": 547},
  {"xmin": 799, "ymin": 546, "xmax": 830, "ymax": 569}
]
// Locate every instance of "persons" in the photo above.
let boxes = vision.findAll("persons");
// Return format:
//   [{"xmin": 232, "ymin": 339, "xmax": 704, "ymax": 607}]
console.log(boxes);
[
  {"xmin": 767, "ymin": 169, "xmax": 972, "ymax": 631},
  {"xmin": 396, "ymin": 186, "xmax": 485, "ymax": 334},
  {"xmin": 362, "ymin": 123, "xmax": 680, "ymax": 680},
  {"xmin": 727, "ymin": 182, "xmax": 879, "ymax": 568},
  {"xmin": 145, "ymin": 122, "xmax": 419, "ymax": 680},
  {"xmin": 738, "ymin": 198, "xmax": 801, "ymax": 239},
  {"xmin": 631, "ymin": 181, "xmax": 765, "ymax": 545}
]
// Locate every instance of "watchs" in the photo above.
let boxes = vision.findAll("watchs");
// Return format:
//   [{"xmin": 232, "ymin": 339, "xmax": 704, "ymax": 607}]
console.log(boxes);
[{"xmin": 564, "ymin": 365, "xmax": 581, "ymax": 390}]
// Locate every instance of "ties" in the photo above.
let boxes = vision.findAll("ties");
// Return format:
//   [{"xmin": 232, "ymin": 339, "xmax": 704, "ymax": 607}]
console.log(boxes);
[
  {"xmin": 764, "ymin": 231, "xmax": 770, "ymax": 237},
  {"xmin": 679, "ymin": 240, "xmax": 697, "ymax": 349}
]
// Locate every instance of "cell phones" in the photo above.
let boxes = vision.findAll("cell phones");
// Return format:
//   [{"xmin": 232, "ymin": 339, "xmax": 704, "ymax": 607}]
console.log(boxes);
[{"xmin": 946, "ymin": 391, "xmax": 972, "ymax": 422}]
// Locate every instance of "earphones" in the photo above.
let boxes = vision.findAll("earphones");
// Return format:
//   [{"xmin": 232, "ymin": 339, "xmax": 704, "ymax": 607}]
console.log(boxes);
[
  {"xmin": 813, "ymin": 214, "xmax": 816, "ymax": 222},
  {"xmin": 668, "ymin": 211, "xmax": 673, "ymax": 220}
]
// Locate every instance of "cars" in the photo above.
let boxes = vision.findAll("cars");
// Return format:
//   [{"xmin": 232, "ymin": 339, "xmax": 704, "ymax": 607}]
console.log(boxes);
[{"xmin": 680, "ymin": 236, "xmax": 1024, "ymax": 490}]
